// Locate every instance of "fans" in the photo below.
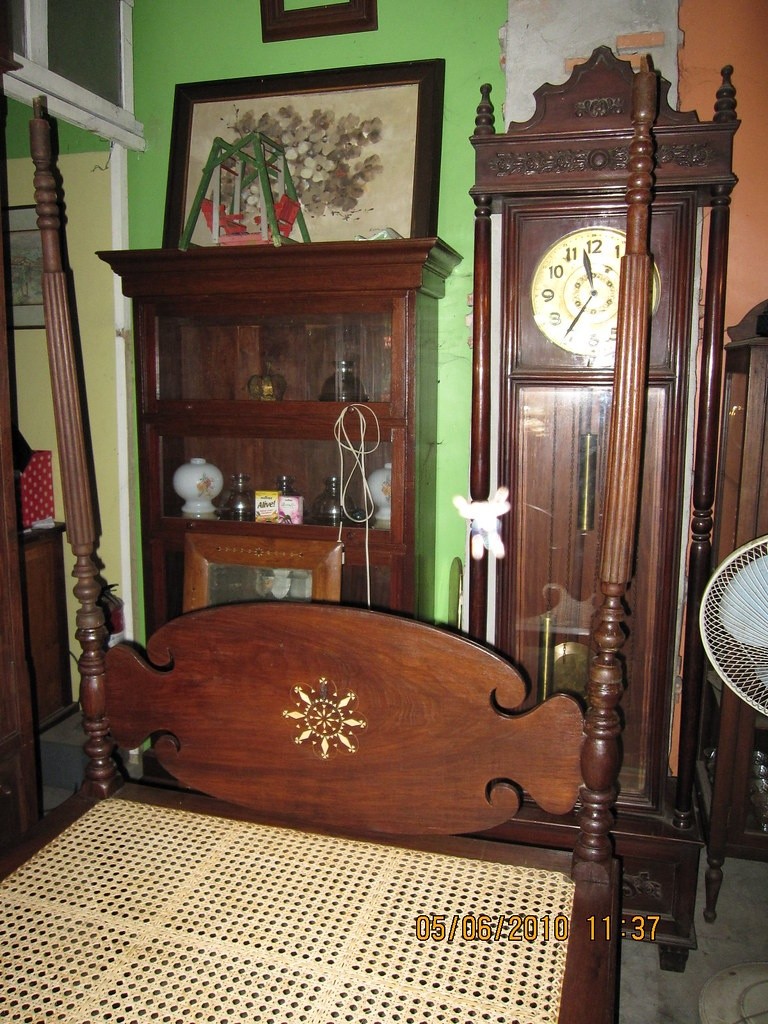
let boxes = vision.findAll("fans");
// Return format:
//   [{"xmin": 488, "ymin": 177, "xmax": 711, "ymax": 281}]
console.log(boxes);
[{"xmin": 698, "ymin": 536, "xmax": 768, "ymax": 1023}]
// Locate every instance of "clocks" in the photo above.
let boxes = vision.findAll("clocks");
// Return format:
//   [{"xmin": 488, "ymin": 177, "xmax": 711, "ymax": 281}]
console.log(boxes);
[{"xmin": 468, "ymin": 46, "xmax": 742, "ymax": 971}]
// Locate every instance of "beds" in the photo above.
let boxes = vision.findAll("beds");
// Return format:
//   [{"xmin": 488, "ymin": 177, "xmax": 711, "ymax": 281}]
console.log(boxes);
[{"xmin": 0, "ymin": 55, "xmax": 668, "ymax": 1024}]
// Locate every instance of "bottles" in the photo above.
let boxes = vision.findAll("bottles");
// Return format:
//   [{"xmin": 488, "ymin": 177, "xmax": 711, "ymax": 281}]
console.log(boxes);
[
  {"xmin": 275, "ymin": 475, "xmax": 300, "ymax": 497},
  {"xmin": 223, "ymin": 473, "xmax": 254, "ymax": 518},
  {"xmin": 317, "ymin": 358, "xmax": 369, "ymax": 402},
  {"xmin": 172, "ymin": 457, "xmax": 222, "ymax": 514},
  {"xmin": 311, "ymin": 475, "xmax": 354, "ymax": 526}
]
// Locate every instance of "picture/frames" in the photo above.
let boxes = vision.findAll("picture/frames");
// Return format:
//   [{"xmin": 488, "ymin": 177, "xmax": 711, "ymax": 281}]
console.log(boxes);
[
  {"xmin": 163, "ymin": 56, "xmax": 447, "ymax": 246},
  {"xmin": 8, "ymin": 205, "xmax": 46, "ymax": 329},
  {"xmin": 260, "ymin": 1, "xmax": 378, "ymax": 43}
]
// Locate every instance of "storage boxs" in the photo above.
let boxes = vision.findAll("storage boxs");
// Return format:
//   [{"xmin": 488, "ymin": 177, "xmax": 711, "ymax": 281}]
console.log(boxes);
[{"xmin": 20, "ymin": 453, "xmax": 54, "ymax": 527}]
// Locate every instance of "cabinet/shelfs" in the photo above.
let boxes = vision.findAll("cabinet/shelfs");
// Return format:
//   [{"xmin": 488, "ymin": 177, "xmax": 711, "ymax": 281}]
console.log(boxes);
[
  {"xmin": 94, "ymin": 237, "xmax": 464, "ymax": 658},
  {"xmin": 675, "ymin": 302, "xmax": 768, "ymax": 922},
  {"xmin": 20, "ymin": 521, "xmax": 80, "ymax": 732}
]
